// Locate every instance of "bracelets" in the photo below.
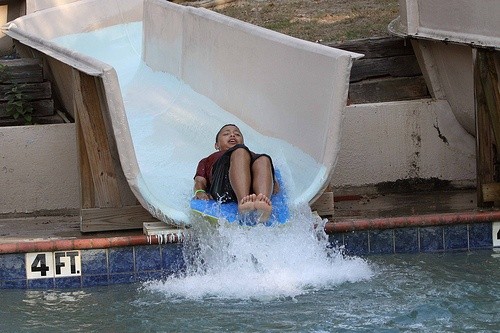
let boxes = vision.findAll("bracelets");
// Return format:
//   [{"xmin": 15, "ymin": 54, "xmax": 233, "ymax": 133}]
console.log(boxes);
[{"xmin": 195, "ymin": 190, "xmax": 206, "ymax": 194}]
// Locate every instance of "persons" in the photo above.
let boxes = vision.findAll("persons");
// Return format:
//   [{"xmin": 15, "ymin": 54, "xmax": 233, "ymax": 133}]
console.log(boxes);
[{"xmin": 194, "ymin": 124, "xmax": 279, "ymax": 223}]
